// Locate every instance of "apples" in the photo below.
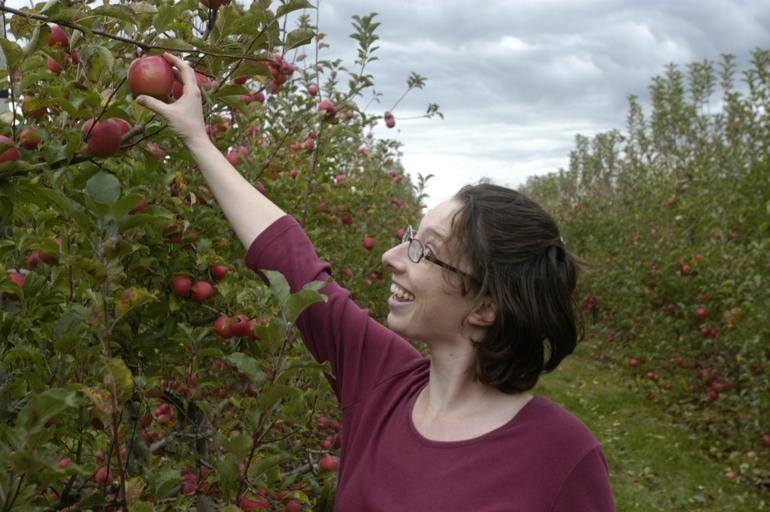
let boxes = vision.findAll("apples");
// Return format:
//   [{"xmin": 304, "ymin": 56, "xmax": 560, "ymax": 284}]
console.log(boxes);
[
  {"xmin": 571, "ymin": 198, "xmax": 770, "ymax": 480},
  {"xmin": 0, "ymin": 22, "xmax": 406, "ymax": 512}
]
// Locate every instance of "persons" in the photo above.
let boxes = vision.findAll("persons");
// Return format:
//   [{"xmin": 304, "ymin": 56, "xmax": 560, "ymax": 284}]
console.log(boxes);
[{"xmin": 133, "ymin": 50, "xmax": 616, "ymax": 511}]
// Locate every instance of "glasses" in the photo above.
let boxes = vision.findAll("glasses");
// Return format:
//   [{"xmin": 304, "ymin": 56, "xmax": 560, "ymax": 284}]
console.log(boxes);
[{"xmin": 401, "ymin": 224, "xmax": 473, "ymax": 282}]
